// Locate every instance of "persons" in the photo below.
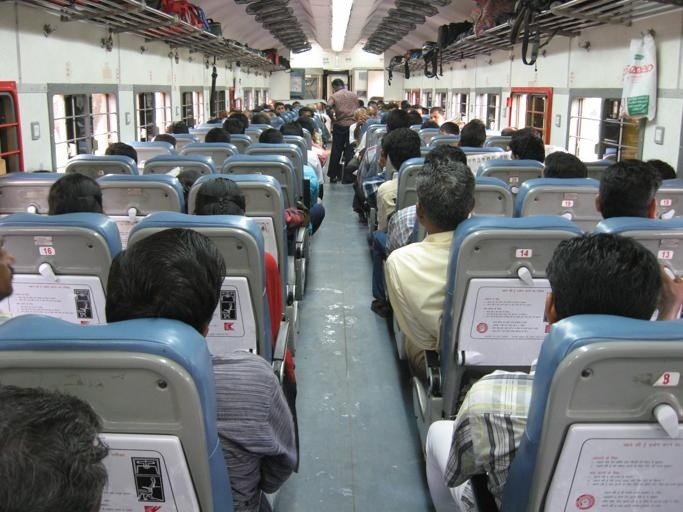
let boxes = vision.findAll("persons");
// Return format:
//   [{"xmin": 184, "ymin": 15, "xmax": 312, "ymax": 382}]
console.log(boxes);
[
  {"xmin": 324, "ymin": 78, "xmax": 359, "ymax": 185},
  {"xmin": 105, "ymin": 101, "xmax": 332, "ymax": 236},
  {"xmin": 194, "ymin": 177, "xmax": 297, "ymax": 406},
  {"xmin": 1, "ymin": 385, "xmax": 109, "ymax": 512},
  {"xmin": 104, "ymin": 228, "xmax": 296, "ymax": 512},
  {"xmin": 48, "ymin": 174, "xmax": 102, "ymax": 215},
  {"xmin": 426, "ymin": 233, "xmax": 683, "ymax": 512},
  {"xmin": 385, "ymin": 163, "xmax": 476, "ymax": 370},
  {"xmin": 596, "ymin": 159, "xmax": 676, "ymax": 220},
  {"xmin": 370, "ymin": 147, "xmax": 466, "ymax": 319},
  {"xmin": 345, "ymin": 96, "xmax": 588, "ymax": 231}
]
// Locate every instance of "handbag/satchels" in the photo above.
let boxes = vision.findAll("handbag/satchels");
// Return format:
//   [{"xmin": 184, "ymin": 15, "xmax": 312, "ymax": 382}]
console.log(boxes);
[
  {"xmin": 206, "ymin": 18, "xmax": 222, "ymax": 38},
  {"xmin": 147, "ymin": 0, "xmax": 211, "ymax": 32}
]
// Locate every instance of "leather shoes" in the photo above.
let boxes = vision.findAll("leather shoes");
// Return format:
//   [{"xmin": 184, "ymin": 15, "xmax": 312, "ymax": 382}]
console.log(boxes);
[
  {"xmin": 330, "ymin": 175, "xmax": 341, "ymax": 183},
  {"xmin": 371, "ymin": 300, "xmax": 392, "ymax": 317}
]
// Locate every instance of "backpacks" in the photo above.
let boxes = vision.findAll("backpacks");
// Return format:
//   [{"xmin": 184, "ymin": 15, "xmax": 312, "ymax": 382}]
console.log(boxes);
[
  {"xmin": 436, "ymin": 19, "xmax": 473, "ymax": 76},
  {"xmin": 421, "ymin": 40, "xmax": 439, "ymax": 80},
  {"xmin": 387, "ymin": 55, "xmax": 405, "ymax": 85},
  {"xmin": 404, "ymin": 48, "xmax": 421, "ymax": 79},
  {"xmin": 493, "ymin": 1, "xmax": 556, "ymax": 66}
]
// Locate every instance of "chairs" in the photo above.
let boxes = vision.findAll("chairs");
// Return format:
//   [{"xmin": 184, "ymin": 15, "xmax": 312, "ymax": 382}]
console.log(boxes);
[
  {"xmin": 0, "ymin": 105, "xmax": 328, "ymax": 392},
  {"xmin": 346, "ymin": 114, "xmax": 682, "ymax": 457},
  {"xmin": 466, "ymin": 313, "xmax": 682, "ymax": 512},
  {"xmin": 1, "ymin": 313, "xmax": 238, "ymax": 512}
]
